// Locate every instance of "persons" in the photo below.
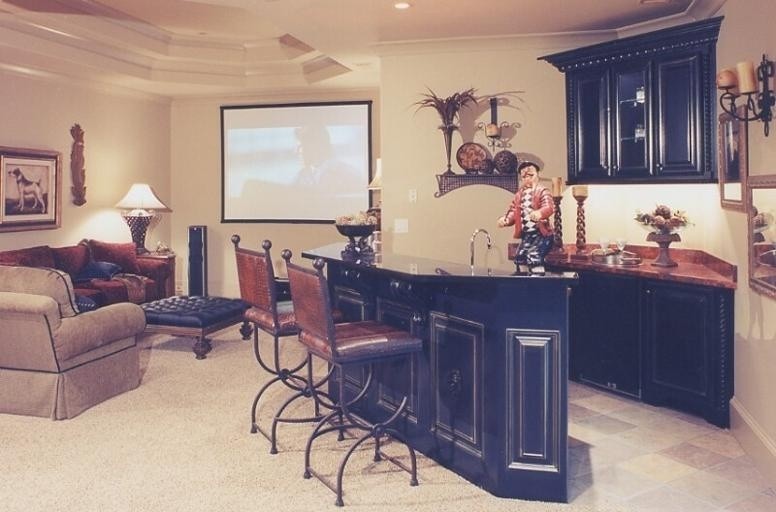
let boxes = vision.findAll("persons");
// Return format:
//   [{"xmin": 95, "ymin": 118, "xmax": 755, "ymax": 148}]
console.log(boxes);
[
  {"xmin": 498, "ymin": 161, "xmax": 554, "ymax": 276},
  {"xmin": 290, "ymin": 124, "xmax": 345, "ymax": 189}
]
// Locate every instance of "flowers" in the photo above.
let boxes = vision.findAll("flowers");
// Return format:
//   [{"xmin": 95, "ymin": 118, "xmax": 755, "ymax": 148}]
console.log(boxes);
[{"xmin": 635, "ymin": 206, "xmax": 688, "ymax": 233}]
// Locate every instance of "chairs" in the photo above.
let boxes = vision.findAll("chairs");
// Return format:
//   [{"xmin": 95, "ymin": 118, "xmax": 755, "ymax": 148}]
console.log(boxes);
[
  {"xmin": 281, "ymin": 249, "xmax": 422, "ymax": 507},
  {"xmin": 231, "ymin": 235, "xmax": 346, "ymax": 454}
]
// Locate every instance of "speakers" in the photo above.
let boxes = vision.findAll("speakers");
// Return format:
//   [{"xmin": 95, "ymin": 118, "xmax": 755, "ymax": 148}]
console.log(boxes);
[{"xmin": 186, "ymin": 225, "xmax": 207, "ymax": 296}]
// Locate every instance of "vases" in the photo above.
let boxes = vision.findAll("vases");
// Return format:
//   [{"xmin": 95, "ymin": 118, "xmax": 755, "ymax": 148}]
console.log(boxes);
[{"xmin": 646, "ymin": 232, "xmax": 681, "ymax": 268}]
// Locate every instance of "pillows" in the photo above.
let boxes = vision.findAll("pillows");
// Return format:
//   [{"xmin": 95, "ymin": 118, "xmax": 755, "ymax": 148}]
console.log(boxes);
[{"xmin": 0, "ymin": 239, "xmax": 141, "ymax": 318}]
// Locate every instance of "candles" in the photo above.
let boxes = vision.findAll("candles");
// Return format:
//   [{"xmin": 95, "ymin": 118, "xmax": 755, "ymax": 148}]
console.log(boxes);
[
  {"xmin": 572, "ymin": 186, "xmax": 589, "ymax": 197},
  {"xmin": 551, "ymin": 176, "xmax": 563, "ymax": 197}
]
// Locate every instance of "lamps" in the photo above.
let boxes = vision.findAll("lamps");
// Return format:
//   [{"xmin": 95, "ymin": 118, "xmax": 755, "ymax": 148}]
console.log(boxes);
[
  {"xmin": 717, "ymin": 53, "xmax": 776, "ymax": 137},
  {"xmin": 368, "ymin": 158, "xmax": 383, "ymax": 214},
  {"xmin": 113, "ymin": 183, "xmax": 168, "ymax": 255},
  {"xmin": 476, "ymin": 97, "xmax": 510, "ymax": 148}
]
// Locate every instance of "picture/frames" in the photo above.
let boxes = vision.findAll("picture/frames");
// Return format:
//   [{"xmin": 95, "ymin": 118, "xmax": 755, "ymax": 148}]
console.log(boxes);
[
  {"xmin": 718, "ymin": 104, "xmax": 749, "ymax": 213},
  {"xmin": 747, "ymin": 174, "xmax": 776, "ymax": 302},
  {"xmin": 0, "ymin": 146, "xmax": 62, "ymax": 233}
]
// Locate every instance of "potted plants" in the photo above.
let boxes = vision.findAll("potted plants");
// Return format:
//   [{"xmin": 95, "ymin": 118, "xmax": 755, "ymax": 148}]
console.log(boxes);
[{"xmin": 412, "ymin": 88, "xmax": 480, "ymax": 175}]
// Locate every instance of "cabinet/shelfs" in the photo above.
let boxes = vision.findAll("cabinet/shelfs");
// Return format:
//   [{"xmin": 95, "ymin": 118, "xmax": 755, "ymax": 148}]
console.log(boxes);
[
  {"xmin": 507, "ymin": 260, "xmax": 737, "ymax": 429},
  {"xmin": 536, "ymin": 15, "xmax": 725, "ymax": 184}
]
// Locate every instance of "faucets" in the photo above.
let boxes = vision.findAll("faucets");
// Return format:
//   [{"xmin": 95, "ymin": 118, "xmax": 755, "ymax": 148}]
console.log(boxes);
[{"xmin": 470, "ymin": 228, "xmax": 492, "ymax": 270}]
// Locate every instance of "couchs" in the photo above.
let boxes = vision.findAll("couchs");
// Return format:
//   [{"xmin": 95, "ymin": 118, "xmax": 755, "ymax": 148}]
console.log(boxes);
[{"xmin": 0, "ymin": 250, "xmax": 171, "ymax": 421}]
